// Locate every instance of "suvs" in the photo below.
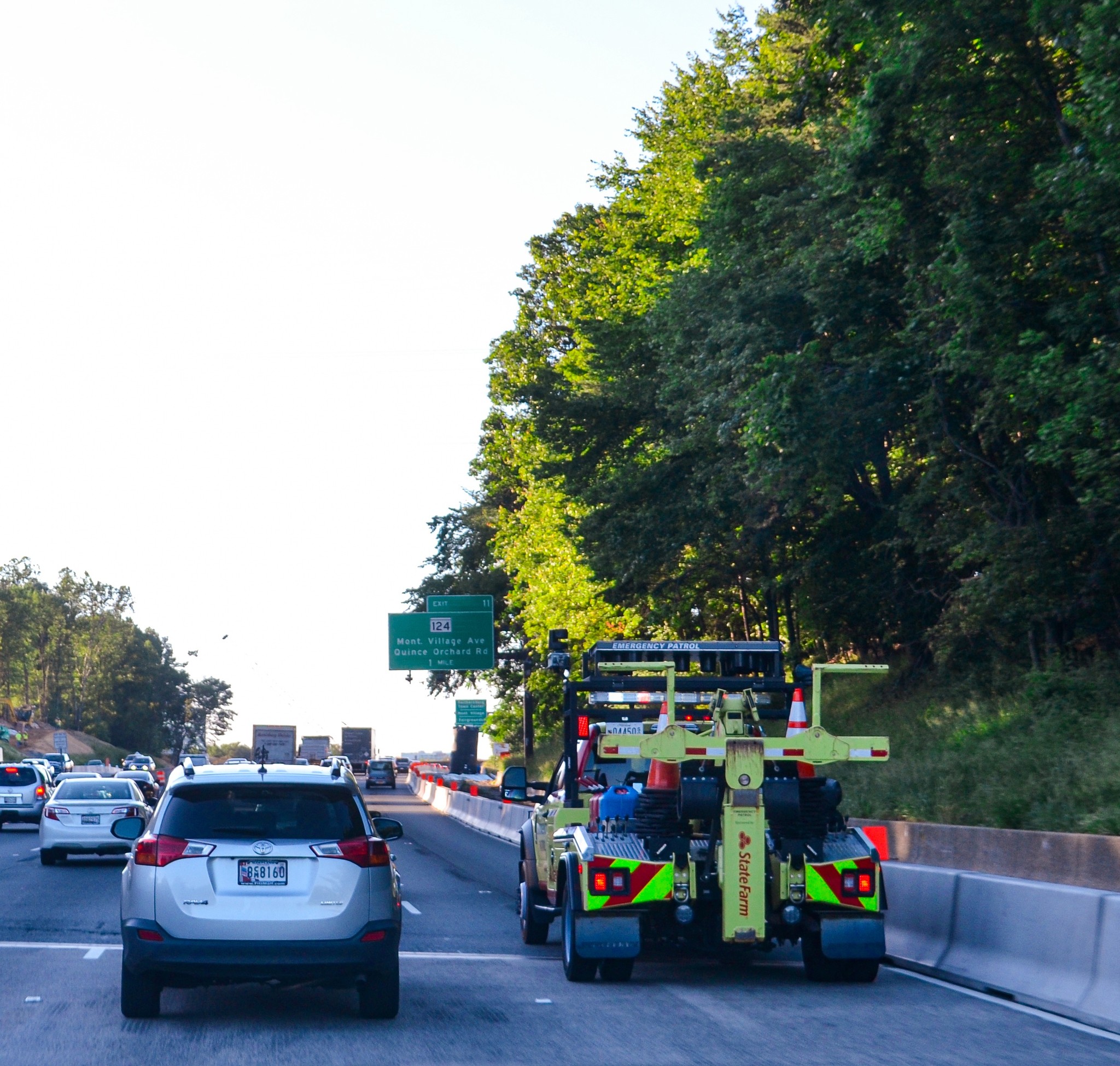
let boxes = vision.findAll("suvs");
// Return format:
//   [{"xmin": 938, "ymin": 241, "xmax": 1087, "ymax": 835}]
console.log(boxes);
[
  {"xmin": 0, "ymin": 763, "xmax": 56, "ymax": 830},
  {"xmin": 110, "ymin": 757, "xmax": 405, "ymax": 1018}
]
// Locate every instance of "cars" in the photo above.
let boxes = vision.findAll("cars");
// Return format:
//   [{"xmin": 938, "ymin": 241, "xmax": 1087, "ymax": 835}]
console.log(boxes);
[
  {"xmin": 294, "ymin": 757, "xmax": 309, "ymax": 766},
  {"xmin": 224, "ymin": 758, "xmax": 248, "ymax": 766},
  {"xmin": 37, "ymin": 777, "xmax": 160, "ymax": 865},
  {"xmin": 43, "ymin": 752, "xmax": 76, "ymax": 776},
  {"xmin": 34, "ymin": 754, "xmax": 162, "ymax": 806},
  {"xmin": 20, "ymin": 758, "xmax": 55, "ymax": 780}
]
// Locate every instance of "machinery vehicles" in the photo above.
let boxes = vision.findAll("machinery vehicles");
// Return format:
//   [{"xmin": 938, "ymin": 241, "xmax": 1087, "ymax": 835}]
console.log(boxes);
[{"xmin": 500, "ymin": 628, "xmax": 890, "ymax": 984}]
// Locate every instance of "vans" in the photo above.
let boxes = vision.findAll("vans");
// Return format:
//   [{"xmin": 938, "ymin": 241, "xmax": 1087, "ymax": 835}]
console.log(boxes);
[
  {"xmin": 321, "ymin": 755, "xmax": 412, "ymax": 789},
  {"xmin": 178, "ymin": 754, "xmax": 210, "ymax": 767}
]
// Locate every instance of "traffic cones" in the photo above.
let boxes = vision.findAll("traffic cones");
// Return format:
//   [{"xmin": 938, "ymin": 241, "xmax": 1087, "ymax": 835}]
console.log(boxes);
[
  {"xmin": 642, "ymin": 700, "xmax": 681, "ymax": 792},
  {"xmin": 782, "ymin": 688, "xmax": 815, "ymax": 778}
]
